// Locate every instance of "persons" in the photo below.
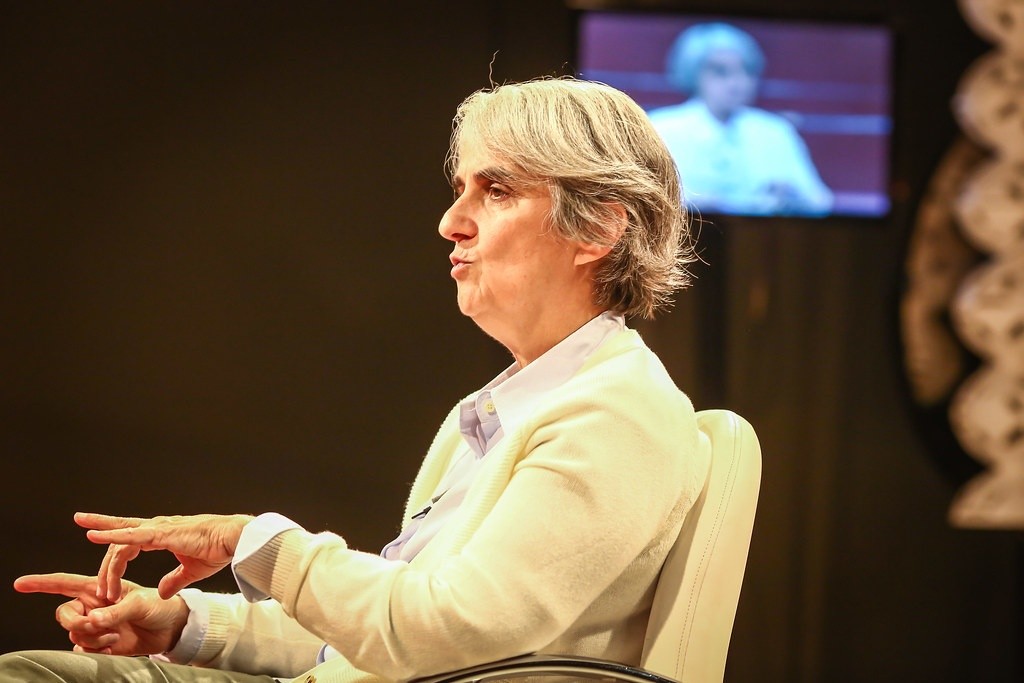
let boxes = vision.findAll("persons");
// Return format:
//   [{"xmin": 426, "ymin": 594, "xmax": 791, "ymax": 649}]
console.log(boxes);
[
  {"xmin": 0, "ymin": 74, "xmax": 714, "ymax": 683},
  {"xmin": 645, "ymin": 21, "xmax": 836, "ymax": 220}
]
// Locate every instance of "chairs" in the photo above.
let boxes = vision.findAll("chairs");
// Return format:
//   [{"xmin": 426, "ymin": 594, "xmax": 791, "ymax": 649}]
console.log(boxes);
[{"xmin": 406, "ymin": 409, "xmax": 762, "ymax": 683}]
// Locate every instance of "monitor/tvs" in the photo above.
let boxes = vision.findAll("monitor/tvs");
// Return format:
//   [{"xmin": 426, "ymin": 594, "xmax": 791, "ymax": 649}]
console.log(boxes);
[{"xmin": 564, "ymin": 7, "xmax": 905, "ymax": 227}]
